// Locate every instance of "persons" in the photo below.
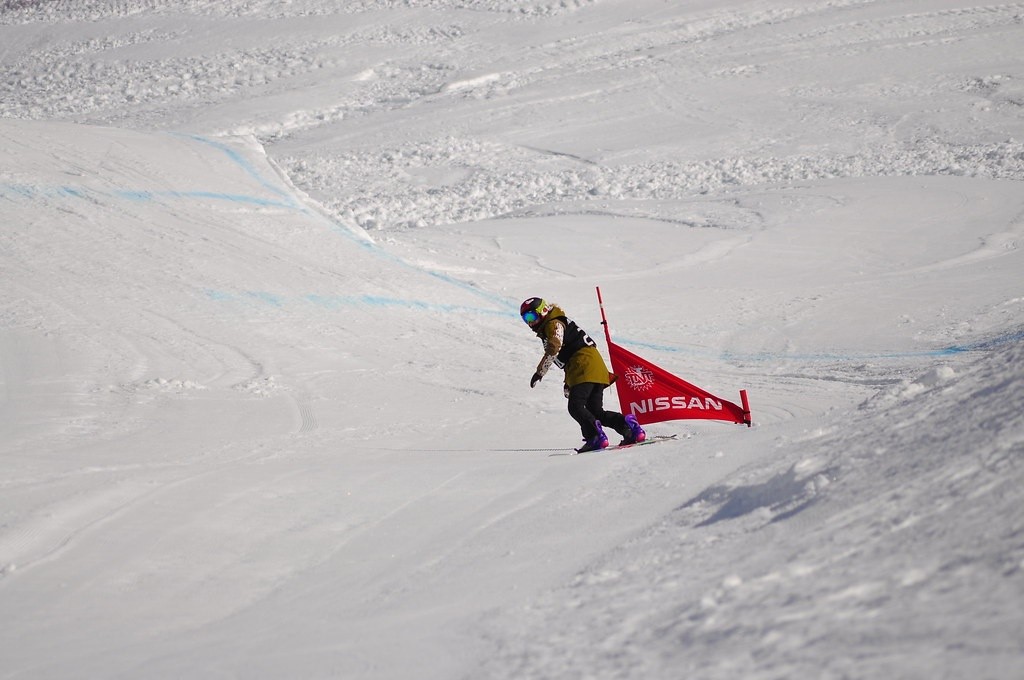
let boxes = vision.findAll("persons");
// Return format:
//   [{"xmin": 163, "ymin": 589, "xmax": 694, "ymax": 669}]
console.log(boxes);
[{"xmin": 520, "ymin": 297, "xmax": 646, "ymax": 453}]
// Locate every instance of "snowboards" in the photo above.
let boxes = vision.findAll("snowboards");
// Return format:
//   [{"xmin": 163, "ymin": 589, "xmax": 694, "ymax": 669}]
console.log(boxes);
[{"xmin": 548, "ymin": 432, "xmax": 678, "ymax": 457}]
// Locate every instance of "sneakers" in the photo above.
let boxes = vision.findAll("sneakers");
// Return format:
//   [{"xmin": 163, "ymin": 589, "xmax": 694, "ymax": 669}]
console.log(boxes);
[
  {"xmin": 619, "ymin": 414, "xmax": 646, "ymax": 446},
  {"xmin": 578, "ymin": 420, "xmax": 609, "ymax": 454}
]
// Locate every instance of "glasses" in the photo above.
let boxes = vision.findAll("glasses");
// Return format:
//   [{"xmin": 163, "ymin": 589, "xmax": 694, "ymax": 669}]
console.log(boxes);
[{"xmin": 523, "ymin": 299, "xmax": 547, "ymax": 324}]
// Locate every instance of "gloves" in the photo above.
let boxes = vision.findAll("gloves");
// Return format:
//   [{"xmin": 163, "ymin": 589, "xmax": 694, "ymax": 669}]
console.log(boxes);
[
  {"xmin": 563, "ymin": 384, "xmax": 571, "ymax": 399},
  {"xmin": 531, "ymin": 372, "xmax": 542, "ymax": 388}
]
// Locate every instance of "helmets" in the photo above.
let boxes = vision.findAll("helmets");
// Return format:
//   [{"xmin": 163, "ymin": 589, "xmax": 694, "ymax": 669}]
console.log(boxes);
[{"xmin": 520, "ymin": 297, "xmax": 549, "ymax": 331}]
